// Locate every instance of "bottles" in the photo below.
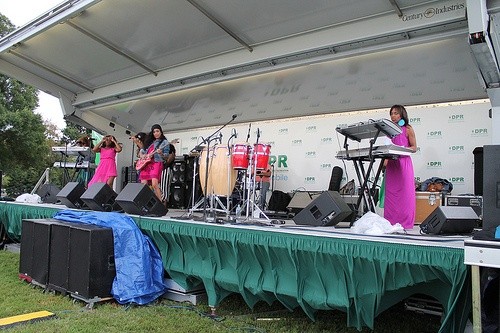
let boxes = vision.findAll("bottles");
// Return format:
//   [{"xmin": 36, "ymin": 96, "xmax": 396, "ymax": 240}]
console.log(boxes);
[{"xmin": 236, "ymin": 204, "xmax": 241, "ymax": 219}]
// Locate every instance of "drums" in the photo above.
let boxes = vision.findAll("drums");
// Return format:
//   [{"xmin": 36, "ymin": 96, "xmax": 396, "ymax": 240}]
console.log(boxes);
[
  {"xmin": 230, "ymin": 142, "xmax": 251, "ymax": 171},
  {"xmin": 199, "ymin": 143, "xmax": 238, "ymax": 197},
  {"xmin": 252, "ymin": 143, "xmax": 272, "ymax": 171}
]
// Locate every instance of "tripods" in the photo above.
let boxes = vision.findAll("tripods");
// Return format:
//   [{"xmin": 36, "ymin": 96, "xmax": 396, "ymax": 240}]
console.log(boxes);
[{"xmin": 188, "ymin": 135, "xmax": 272, "ymax": 223}]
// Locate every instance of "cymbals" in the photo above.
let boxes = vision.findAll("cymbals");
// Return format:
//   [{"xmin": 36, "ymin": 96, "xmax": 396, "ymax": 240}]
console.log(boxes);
[{"xmin": 253, "ymin": 172, "xmax": 269, "ymax": 176}]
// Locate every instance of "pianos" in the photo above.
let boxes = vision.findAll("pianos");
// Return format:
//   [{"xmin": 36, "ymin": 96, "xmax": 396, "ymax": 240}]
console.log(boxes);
[
  {"xmin": 332, "ymin": 118, "xmax": 416, "ymax": 230},
  {"xmin": 48, "ymin": 146, "xmax": 97, "ymax": 189}
]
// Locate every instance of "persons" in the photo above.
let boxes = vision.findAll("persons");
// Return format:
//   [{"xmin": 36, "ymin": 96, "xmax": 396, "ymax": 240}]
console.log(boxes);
[
  {"xmin": 74, "ymin": 124, "xmax": 176, "ymax": 207},
  {"xmin": 248, "ymin": 165, "xmax": 271, "ymax": 212},
  {"xmin": 381, "ymin": 104, "xmax": 418, "ymax": 230}
]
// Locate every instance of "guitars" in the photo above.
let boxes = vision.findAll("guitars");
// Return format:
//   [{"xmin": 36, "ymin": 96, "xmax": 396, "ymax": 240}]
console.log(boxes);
[{"xmin": 135, "ymin": 138, "xmax": 180, "ymax": 171}]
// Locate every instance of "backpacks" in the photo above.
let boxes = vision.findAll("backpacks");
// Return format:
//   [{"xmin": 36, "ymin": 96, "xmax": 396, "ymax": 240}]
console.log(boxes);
[{"xmin": 162, "ymin": 144, "xmax": 175, "ymax": 168}]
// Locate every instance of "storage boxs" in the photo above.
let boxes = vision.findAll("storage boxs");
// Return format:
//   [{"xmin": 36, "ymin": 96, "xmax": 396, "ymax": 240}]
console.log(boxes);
[
  {"xmin": 445, "ymin": 196, "xmax": 483, "ymax": 217},
  {"xmin": 336, "ymin": 195, "xmax": 372, "ymax": 227},
  {"xmin": 414, "ymin": 191, "xmax": 442, "ymax": 225}
]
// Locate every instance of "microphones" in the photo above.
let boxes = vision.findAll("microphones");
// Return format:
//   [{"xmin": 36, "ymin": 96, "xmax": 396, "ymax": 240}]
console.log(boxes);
[
  {"xmin": 234, "ymin": 129, "xmax": 237, "ymax": 138},
  {"xmin": 219, "ymin": 132, "xmax": 221, "ymax": 144},
  {"xmin": 258, "ymin": 128, "xmax": 260, "ymax": 137},
  {"xmin": 248, "ymin": 124, "xmax": 251, "ymax": 138},
  {"xmin": 88, "ymin": 137, "xmax": 96, "ymax": 140}
]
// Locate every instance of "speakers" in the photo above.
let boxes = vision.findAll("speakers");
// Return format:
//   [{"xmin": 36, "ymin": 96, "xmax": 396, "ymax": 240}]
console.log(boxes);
[
  {"xmin": 287, "ymin": 191, "xmax": 373, "ymax": 226},
  {"xmin": 37, "ymin": 181, "xmax": 169, "ymax": 221},
  {"xmin": 169, "ymin": 217, "xmax": 170, "ymax": 219},
  {"xmin": 19, "ymin": 218, "xmax": 118, "ymax": 298},
  {"xmin": 420, "ymin": 206, "xmax": 481, "ymax": 235}
]
